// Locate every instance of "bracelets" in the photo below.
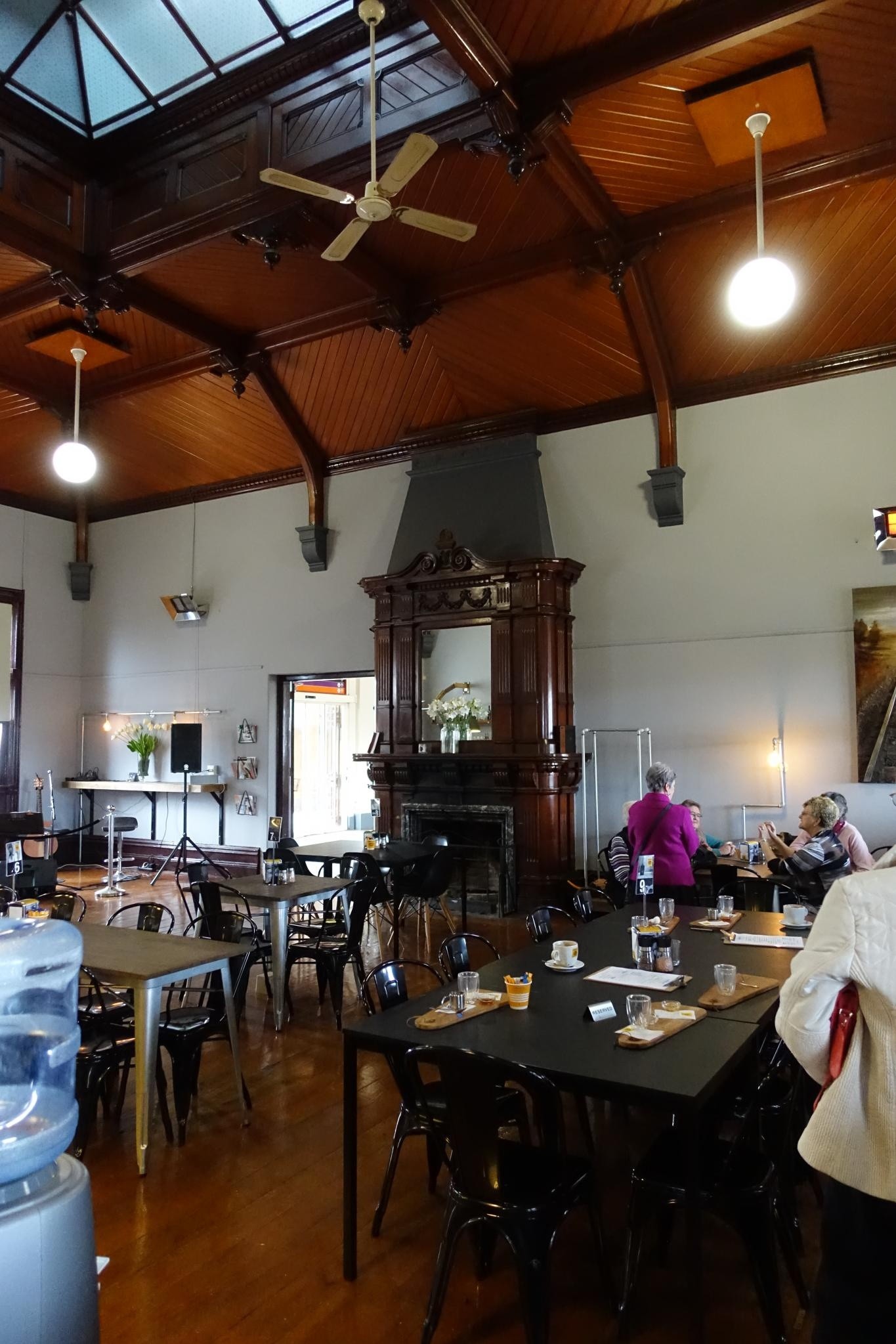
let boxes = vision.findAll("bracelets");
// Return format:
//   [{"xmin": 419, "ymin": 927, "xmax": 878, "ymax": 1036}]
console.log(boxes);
[{"xmin": 759, "ymin": 837, "xmax": 767, "ymax": 843}]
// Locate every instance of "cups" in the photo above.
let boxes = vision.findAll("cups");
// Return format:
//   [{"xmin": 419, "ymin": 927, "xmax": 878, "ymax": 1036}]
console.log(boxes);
[
  {"xmin": 631, "ymin": 915, "xmax": 649, "ymax": 965},
  {"xmin": 440, "ymin": 990, "xmax": 466, "ymax": 1009},
  {"xmin": 626, "ymin": 993, "xmax": 652, "ymax": 1031},
  {"xmin": 716, "ymin": 894, "xmax": 734, "ymax": 919},
  {"xmin": 783, "ymin": 903, "xmax": 808, "ymax": 924},
  {"xmin": 707, "ymin": 908, "xmax": 718, "ymax": 920},
  {"xmin": 658, "ymin": 897, "xmax": 674, "ymax": 924},
  {"xmin": 504, "ymin": 975, "xmax": 532, "ymax": 1010},
  {"xmin": 551, "ymin": 940, "xmax": 578, "ymax": 967},
  {"xmin": 714, "ymin": 964, "xmax": 737, "ymax": 996},
  {"xmin": 457, "ymin": 971, "xmax": 480, "ymax": 1004},
  {"xmin": 670, "ymin": 938, "xmax": 681, "ymax": 966},
  {"xmin": 755, "ymin": 847, "xmax": 766, "ymax": 866}
]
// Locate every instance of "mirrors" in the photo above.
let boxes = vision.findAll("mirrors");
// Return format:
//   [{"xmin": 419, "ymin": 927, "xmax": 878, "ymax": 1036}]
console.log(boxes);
[
  {"xmin": 0, "ymin": 601, "xmax": 15, "ymax": 784},
  {"xmin": 418, "ymin": 620, "xmax": 493, "ymax": 743}
]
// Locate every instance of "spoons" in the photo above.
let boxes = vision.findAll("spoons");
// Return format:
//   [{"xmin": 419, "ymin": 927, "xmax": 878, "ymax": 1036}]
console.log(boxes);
[
  {"xmin": 428, "ymin": 1006, "xmax": 467, "ymax": 1013},
  {"xmin": 542, "ymin": 959, "xmax": 575, "ymax": 968}
]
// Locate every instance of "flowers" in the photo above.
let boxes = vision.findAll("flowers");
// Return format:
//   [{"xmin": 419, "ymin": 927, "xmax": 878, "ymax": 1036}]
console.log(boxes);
[
  {"xmin": 425, "ymin": 698, "xmax": 470, "ymax": 723},
  {"xmin": 109, "ymin": 718, "xmax": 170, "ymax": 773},
  {"xmin": 458, "ymin": 697, "xmax": 489, "ymax": 739}
]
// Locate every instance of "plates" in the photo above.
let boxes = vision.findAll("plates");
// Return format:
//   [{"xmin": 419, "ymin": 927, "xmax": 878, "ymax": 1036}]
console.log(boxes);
[
  {"xmin": 661, "ymin": 999, "xmax": 681, "ymax": 1010},
  {"xmin": 545, "ymin": 959, "xmax": 584, "ymax": 972},
  {"xmin": 779, "ymin": 920, "xmax": 812, "ymax": 930}
]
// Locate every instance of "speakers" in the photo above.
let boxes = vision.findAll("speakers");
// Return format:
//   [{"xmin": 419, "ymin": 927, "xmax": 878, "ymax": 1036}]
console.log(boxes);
[{"xmin": 170, "ymin": 723, "xmax": 203, "ymax": 773}]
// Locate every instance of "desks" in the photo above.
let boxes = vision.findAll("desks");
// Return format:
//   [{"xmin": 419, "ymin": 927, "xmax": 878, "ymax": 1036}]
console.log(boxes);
[{"xmin": 58, "ymin": 777, "xmax": 227, "ymax": 845}]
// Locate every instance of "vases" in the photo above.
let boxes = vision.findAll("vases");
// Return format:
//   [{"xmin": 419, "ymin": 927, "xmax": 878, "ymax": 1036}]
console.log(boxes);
[
  {"xmin": 440, "ymin": 723, "xmax": 460, "ymax": 753},
  {"xmin": 456, "ymin": 722, "xmax": 472, "ymax": 741},
  {"xmin": 137, "ymin": 751, "xmax": 150, "ymax": 782}
]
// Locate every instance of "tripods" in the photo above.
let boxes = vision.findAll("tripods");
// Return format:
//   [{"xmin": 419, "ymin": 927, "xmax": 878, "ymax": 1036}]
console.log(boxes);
[{"xmin": 149, "ymin": 773, "xmax": 233, "ymax": 885}]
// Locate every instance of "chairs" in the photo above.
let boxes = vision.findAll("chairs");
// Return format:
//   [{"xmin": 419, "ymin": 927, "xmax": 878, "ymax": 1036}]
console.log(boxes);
[{"xmin": 0, "ymin": 819, "xmax": 893, "ymax": 1344}]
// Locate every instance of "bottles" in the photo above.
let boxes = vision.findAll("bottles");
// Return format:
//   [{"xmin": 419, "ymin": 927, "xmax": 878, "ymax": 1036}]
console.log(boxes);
[
  {"xmin": 277, "ymin": 859, "xmax": 294, "ymax": 885},
  {"xmin": 0, "ymin": 915, "xmax": 85, "ymax": 1187},
  {"xmin": 372, "ymin": 832, "xmax": 388, "ymax": 849},
  {"xmin": 655, "ymin": 934, "xmax": 673, "ymax": 973},
  {"xmin": 636, "ymin": 932, "xmax": 657, "ymax": 972}
]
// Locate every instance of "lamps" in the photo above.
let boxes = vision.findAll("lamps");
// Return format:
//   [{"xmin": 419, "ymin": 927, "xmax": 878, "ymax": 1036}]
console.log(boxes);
[
  {"xmin": 872, "ymin": 506, "xmax": 896, "ymax": 551},
  {"xmin": 726, "ymin": 112, "xmax": 799, "ymax": 326},
  {"xmin": 158, "ymin": 593, "xmax": 207, "ymax": 622},
  {"xmin": 52, "ymin": 348, "xmax": 98, "ymax": 484}
]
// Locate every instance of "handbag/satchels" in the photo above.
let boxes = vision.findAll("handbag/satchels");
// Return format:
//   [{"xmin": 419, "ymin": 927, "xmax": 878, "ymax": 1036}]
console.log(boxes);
[{"xmin": 809, "ymin": 982, "xmax": 859, "ymax": 1111}]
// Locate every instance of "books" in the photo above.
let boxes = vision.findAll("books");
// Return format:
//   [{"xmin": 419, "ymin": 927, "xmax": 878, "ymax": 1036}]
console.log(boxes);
[
  {"xmin": 236, "ymin": 724, "xmax": 257, "ymax": 743},
  {"xmin": 232, "ymin": 755, "xmax": 257, "ymax": 780},
  {"xmin": 235, "ymin": 794, "xmax": 257, "ymax": 815}
]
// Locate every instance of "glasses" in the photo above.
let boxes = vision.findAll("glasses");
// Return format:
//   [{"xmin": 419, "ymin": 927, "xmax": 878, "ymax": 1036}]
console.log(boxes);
[
  {"xmin": 689, "ymin": 811, "xmax": 702, "ymax": 818},
  {"xmin": 801, "ymin": 811, "xmax": 817, "ymax": 818}
]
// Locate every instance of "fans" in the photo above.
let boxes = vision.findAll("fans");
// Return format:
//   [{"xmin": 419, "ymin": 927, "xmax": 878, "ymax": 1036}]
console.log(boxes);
[{"xmin": 257, "ymin": 0, "xmax": 480, "ymax": 263}]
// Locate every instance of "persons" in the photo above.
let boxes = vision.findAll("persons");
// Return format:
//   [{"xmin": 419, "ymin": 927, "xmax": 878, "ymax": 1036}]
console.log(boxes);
[
  {"xmin": 604, "ymin": 799, "xmax": 639, "ymax": 910},
  {"xmin": 788, "ymin": 790, "xmax": 876, "ymax": 873},
  {"xmin": 679, "ymin": 797, "xmax": 736, "ymax": 860},
  {"xmin": 6, "ymin": 844, "xmax": 18, "ymax": 862},
  {"xmin": 627, "ymin": 761, "xmax": 701, "ymax": 907},
  {"xmin": 757, "ymin": 796, "xmax": 851, "ymax": 914},
  {"xmin": 771, "ymin": 844, "xmax": 896, "ymax": 1344}
]
